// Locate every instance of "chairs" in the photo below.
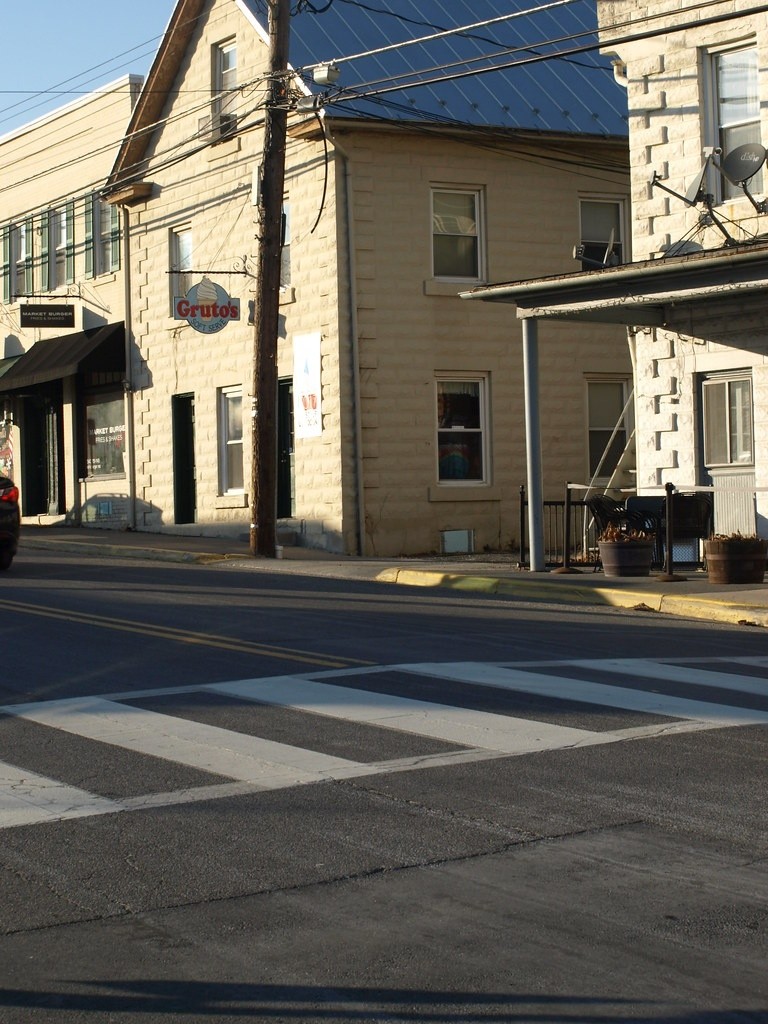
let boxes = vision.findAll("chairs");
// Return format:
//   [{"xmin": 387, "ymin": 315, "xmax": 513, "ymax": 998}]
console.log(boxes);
[{"xmin": 585, "ymin": 487, "xmax": 717, "ymax": 576}]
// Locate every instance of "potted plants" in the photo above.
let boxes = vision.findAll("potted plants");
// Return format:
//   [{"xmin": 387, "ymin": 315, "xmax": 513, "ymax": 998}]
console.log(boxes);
[
  {"xmin": 704, "ymin": 532, "xmax": 768, "ymax": 585},
  {"xmin": 598, "ymin": 522, "xmax": 658, "ymax": 575}
]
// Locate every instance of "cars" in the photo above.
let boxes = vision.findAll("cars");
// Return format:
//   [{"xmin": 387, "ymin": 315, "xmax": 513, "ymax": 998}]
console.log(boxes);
[{"xmin": 0, "ymin": 470, "xmax": 20, "ymax": 569}]
